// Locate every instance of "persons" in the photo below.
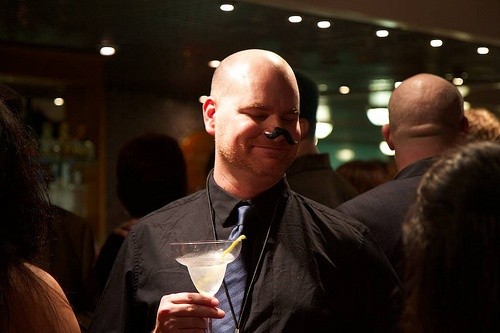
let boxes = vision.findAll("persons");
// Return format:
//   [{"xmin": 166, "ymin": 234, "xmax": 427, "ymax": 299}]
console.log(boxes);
[
  {"xmin": 396, "ymin": 141, "xmax": 500, "ymax": 333},
  {"xmin": 0, "ymin": 99, "xmax": 82, "ymax": 333},
  {"xmin": 77, "ymin": 73, "xmax": 500, "ymax": 333},
  {"xmin": 88, "ymin": 48, "xmax": 400, "ymax": 332}
]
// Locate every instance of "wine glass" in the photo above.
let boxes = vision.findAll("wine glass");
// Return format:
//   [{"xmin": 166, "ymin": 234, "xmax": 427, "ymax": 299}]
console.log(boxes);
[{"xmin": 171, "ymin": 240, "xmax": 243, "ymax": 333}]
